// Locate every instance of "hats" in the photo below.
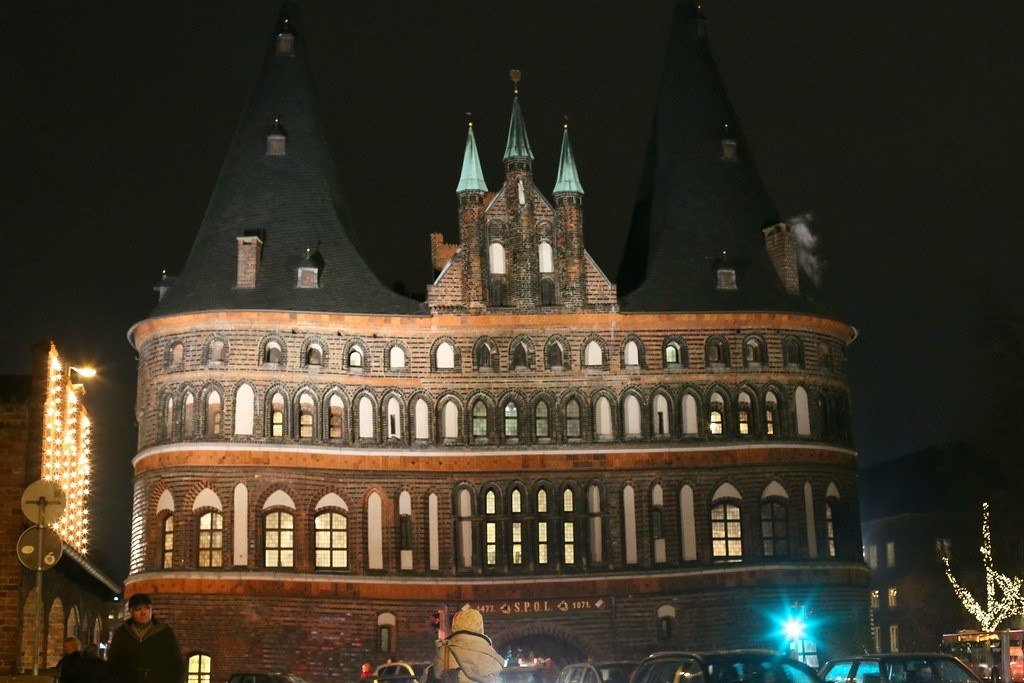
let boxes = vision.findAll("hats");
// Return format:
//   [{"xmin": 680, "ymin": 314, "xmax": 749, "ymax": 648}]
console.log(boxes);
[
  {"xmin": 128, "ymin": 594, "xmax": 151, "ymax": 608},
  {"xmin": 452, "ymin": 609, "xmax": 484, "ymax": 635}
]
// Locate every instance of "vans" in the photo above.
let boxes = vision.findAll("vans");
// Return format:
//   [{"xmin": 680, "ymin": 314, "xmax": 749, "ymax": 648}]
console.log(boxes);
[
  {"xmin": 501, "ymin": 667, "xmax": 560, "ymax": 683},
  {"xmin": 375, "ymin": 663, "xmax": 433, "ymax": 683}
]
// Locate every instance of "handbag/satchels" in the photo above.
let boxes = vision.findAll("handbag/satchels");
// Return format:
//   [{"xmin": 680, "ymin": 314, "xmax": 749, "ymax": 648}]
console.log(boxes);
[{"xmin": 432, "ymin": 668, "xmax": 459, "ymax": 683}]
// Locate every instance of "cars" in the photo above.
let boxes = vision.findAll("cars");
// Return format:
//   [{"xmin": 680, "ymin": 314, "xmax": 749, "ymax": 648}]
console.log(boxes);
[
  {"xmin": 559, "ymin": 649, "xmax": 982, "ymax": 683},
  {"xmin": 225, "ymin": 671, "xmax": 306, "ymax": 683}
]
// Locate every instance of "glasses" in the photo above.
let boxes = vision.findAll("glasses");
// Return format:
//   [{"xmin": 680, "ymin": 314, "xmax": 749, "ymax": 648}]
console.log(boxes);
[{"xmin": 132, "ymin": 605, "xmax": 153, "ymax": 614}]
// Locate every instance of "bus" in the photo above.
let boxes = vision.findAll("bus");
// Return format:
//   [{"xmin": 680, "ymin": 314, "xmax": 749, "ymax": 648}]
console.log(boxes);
[{"xmin": 944, "ymin": 630, "xmax": 1024, "ymax": 683}]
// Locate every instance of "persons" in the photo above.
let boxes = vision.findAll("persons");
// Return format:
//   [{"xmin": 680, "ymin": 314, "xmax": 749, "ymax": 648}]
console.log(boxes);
[
  {"xmin": 629, "ymin": 668, "xmax": 641, "ymax": 682},
  {"xmin": 912, "ymin": 662, "xmax": 935, "ymax": 683},
  {"xmin": 761, "ymin": 668, "xmax": 778, "ymax": 683},
  {"xmin": 431, "ymin": 608, "xmax": 507, "ymax": 683},
  {"xmin": 105, "ymin": 594, "xmax": 190, "ymax": 682},
  {"xmin": 73, "ymin": 644, "xmax": 106, "ymax": 682},
  {"xmin": 53, "ymin": 635, "xmax": 83, "ymax": 683}
]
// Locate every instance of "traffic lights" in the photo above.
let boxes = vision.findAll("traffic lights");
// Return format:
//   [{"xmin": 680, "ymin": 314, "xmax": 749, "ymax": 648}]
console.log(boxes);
[{"xmin": 432, "ymin": 610, "xmax": 444, "ymax": 630}]
